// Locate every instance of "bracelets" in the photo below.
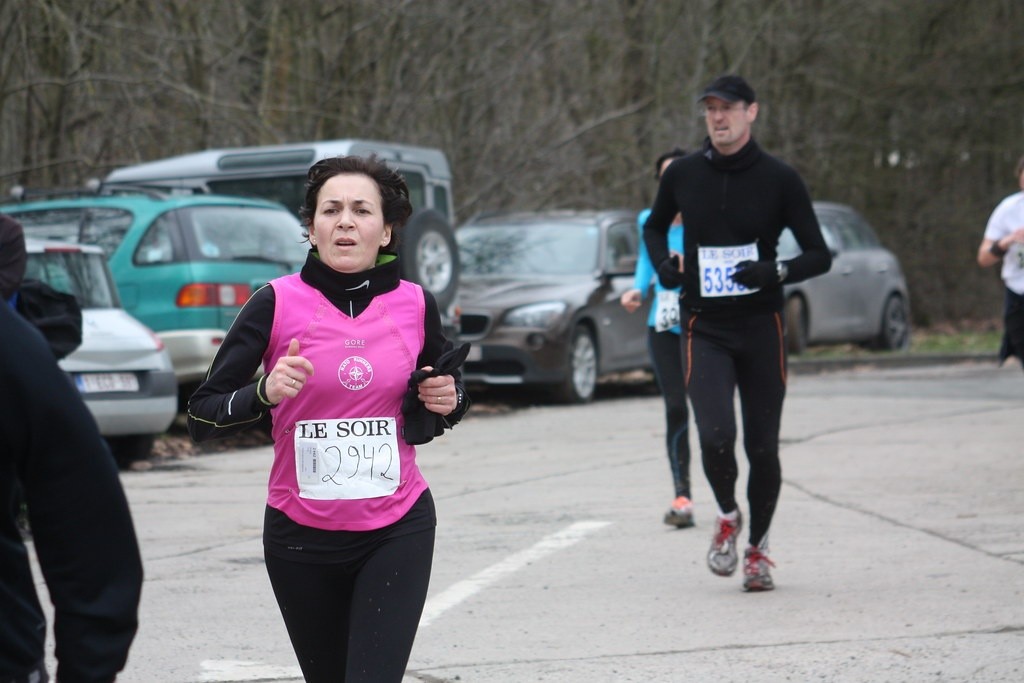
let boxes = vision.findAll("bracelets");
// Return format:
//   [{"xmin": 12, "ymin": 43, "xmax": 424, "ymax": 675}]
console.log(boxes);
[
  {"xmin": 989, "ymin": 239, "xmax": 1008, "ymax": 257},
  {"xmin": 776, "ymin": 260, "xmax": 788, "ymax": 283}
]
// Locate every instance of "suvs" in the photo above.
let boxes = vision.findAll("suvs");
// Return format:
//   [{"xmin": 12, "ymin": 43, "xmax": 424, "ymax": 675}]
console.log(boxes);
[{"xmin": 78, "ymin": 139, "xmax": 462, "ymax": 348}]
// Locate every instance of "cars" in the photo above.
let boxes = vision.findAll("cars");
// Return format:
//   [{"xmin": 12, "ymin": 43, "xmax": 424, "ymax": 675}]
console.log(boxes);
[
  {"xmin": 774, "ymin": 202, "xmax": 912, "ymax": 355},
  {"xmin": 19, "ymin": 238, "xmax": 180, "ymax": 474},
  {"xmin": 0, "ymin": 193, "xmax": 313, "ymax": 382},
  {"xmin": 443, "ymin": 208, "xmax": 654, "ymax": 406}
]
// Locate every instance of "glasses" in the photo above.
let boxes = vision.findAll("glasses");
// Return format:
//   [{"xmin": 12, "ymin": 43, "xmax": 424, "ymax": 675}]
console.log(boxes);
[{"xmin": 701, "ymin": 105, "xmax": 747, "ymax": 115}]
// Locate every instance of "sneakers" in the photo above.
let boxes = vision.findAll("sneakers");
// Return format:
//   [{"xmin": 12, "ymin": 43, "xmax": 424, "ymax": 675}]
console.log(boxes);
[
  {"xmin": 743, "ymin": 547, "xmax": 774, "ymax": 589},
  {"xmin": 708, "ymin": 514, "xmax": 740, "ymax": 574}
]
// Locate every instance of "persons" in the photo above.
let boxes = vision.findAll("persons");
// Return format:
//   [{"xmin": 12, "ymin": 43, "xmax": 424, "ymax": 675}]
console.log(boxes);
[
  {"xmin": 976, "ymin": 162, "xmax": 1024, "ymax": 376},
  {"xmin": 620, "ymin": 147, "xmax": 693, "ymax": 527},
  {"xmin": 188, "ymin": 155, "xmax": 470, "ymax": 683},
  {"xmin": 0, "ymin": 213, "xmax": 144, "ymax": 683},
  {"xmin": 643, "ymin": 75, "xmax": 832, "ymax": 591}
]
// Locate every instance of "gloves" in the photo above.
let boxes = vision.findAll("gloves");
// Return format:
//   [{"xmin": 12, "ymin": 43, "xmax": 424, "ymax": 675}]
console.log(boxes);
[
  {"xmin": 731, "ymin": 260, "xmax": 779, "ymax": 288},
  {"xmin": 660, "ymin": 255, "xmax": 686, "ymax": 288}
]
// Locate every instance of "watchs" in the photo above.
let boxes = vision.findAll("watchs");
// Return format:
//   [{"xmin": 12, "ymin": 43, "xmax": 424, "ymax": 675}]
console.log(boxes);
[{"xmin": 452, "ymin": 387, "xmax": 464, "ymax": 414}]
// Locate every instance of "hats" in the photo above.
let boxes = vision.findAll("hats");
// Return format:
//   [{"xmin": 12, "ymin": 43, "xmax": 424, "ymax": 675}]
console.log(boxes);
[{"xmin": 696, "ymin": 75, "xmax": 754, "ymax": 106}]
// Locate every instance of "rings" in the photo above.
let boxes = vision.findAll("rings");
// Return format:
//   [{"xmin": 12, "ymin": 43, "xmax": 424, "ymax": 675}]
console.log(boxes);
[
  {"xmin": 437, "ymin": 397, "xmax": 441, "ymax": 404},
  {"xmin": 292, "ymin": 379, "xmax": 296, "ymax": 386}
]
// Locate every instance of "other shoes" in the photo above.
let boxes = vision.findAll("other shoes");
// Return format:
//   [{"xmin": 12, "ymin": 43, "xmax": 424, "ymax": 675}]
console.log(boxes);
[{"xmin": 665, "ymin": 505, "xmax": 694, "ymax": 527}]
